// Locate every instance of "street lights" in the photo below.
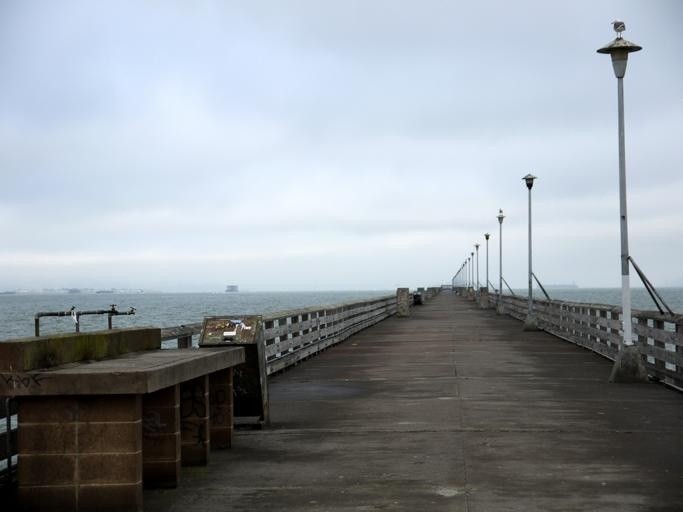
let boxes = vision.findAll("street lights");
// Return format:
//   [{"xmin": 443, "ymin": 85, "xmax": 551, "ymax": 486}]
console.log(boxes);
[
  {"xmin": 492, "ymin": 208, "xmax": 507, "ymax": 316},
  {"xmin": 522, "ymin": 172, "xmax": 540, "ymax": 333},
  {"xmin": 451, "ymin": 244, "xmax": 480, "ymax": 291},
  {"xmin": 483, "ymin": 232, "xmax": 490, "ymax": 294},
  {"xmin": 594, "ymin": 18, "xmax": 650, "ymax": 384}
]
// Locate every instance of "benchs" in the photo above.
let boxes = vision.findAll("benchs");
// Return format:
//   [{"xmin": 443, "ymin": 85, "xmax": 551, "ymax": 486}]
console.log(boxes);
[{"xmin": 0, "ymin": 324, "xmax": 245, "ymax": 511}]
